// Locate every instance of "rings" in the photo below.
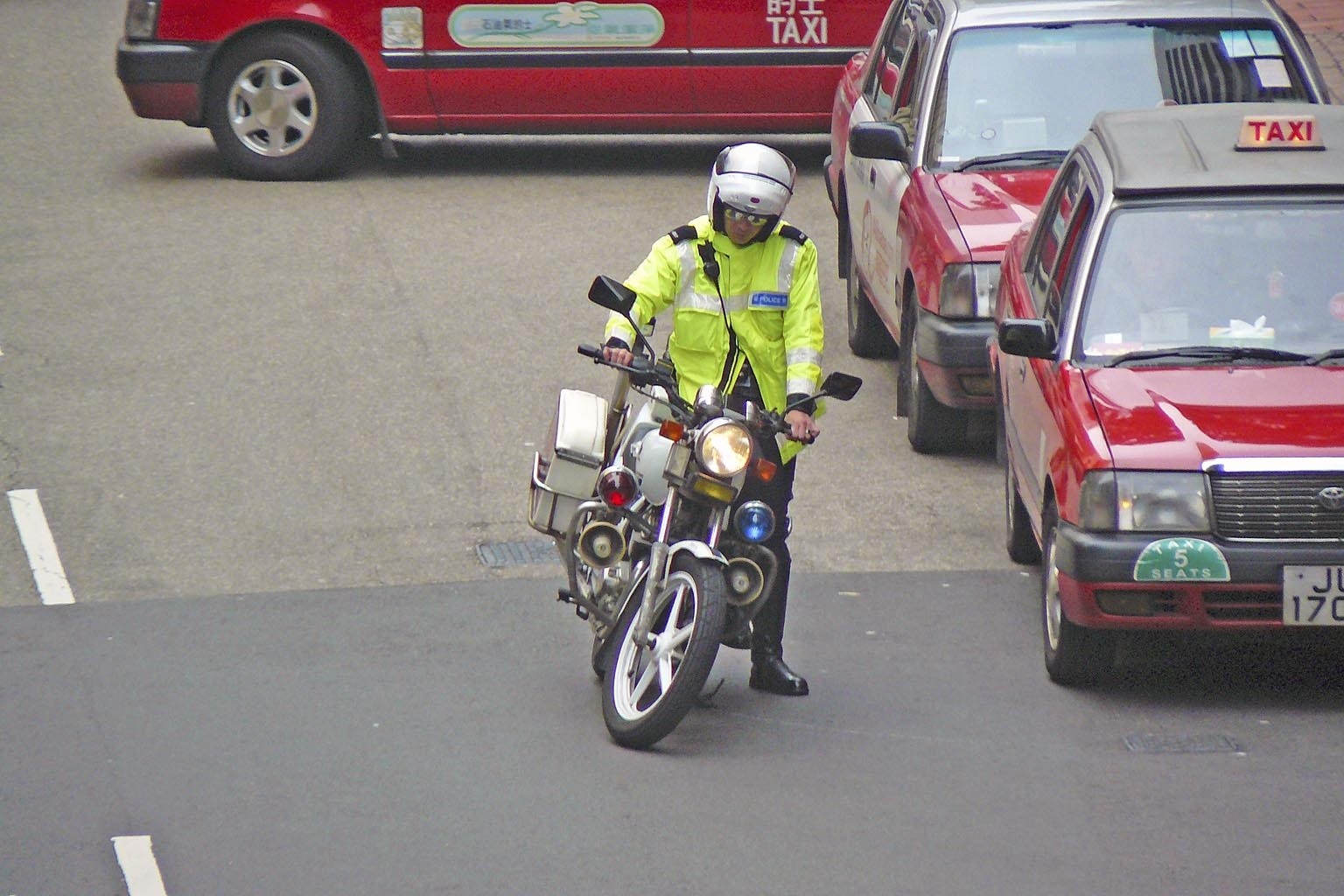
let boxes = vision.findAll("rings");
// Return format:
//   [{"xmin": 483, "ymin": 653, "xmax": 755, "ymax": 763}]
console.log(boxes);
[{"xmin": 806, "ymin": 430, "xmax": 810, "ymax": 434}]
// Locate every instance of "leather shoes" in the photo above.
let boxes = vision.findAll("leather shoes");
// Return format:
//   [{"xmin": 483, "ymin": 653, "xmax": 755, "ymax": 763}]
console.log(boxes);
[
  {"xmin": 721, "ymin": 606, "xmax": 754, "ymax": 650},
  {"xmin": 749, "ymin": 643, "xmax": 809, "ymax": 696}
]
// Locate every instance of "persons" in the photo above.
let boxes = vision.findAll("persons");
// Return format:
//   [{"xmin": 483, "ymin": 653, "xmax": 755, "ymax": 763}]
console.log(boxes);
[{"xmin": 603, "ymin": 141, "xmax": 827, "ymax": 696}]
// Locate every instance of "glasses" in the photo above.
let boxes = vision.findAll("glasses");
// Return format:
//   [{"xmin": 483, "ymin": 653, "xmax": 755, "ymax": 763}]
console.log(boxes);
[{"xmin": 721, "ymin": 205, "xmax": 771, "ymax": 226}]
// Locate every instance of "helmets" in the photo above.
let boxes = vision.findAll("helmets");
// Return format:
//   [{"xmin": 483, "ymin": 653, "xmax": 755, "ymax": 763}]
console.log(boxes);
[{"xmin": 706, "ymin": 141, "xmax": 796, "ymax": 243}]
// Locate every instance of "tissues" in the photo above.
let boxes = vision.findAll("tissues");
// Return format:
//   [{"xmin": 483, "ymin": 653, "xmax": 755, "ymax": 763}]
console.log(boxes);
[{"xmin": 1208, "ymin": 314, "xmax": 1276, "ymax": 349}]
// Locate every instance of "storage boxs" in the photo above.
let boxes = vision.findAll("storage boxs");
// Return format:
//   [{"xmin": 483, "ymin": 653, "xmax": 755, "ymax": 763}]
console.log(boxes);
[{"xmin": 535, "ymin": 388, "xmax": 609, "ymax": 533}]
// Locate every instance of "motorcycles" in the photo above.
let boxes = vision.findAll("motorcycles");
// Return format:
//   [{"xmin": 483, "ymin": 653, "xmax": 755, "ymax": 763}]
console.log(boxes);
[{"xmin": 525, "ymin": 274, "xmax": 867, "ymax": 752}]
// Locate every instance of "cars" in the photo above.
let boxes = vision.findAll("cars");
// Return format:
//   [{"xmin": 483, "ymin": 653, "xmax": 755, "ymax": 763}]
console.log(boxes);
[
  {"xmin": 113, "ymin": 0, "xmax": 901, "ymax": 185},
  {"xmin": 989, "ymin": 101, "xmax": 1344, "ymax": 689},
  {"xmin": 821, "ymin": 2, "xmax": 1342, "ymax": 458}
]
